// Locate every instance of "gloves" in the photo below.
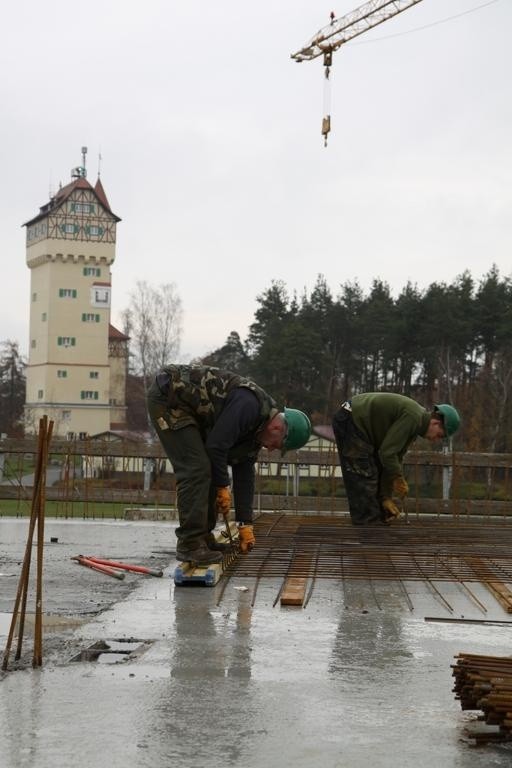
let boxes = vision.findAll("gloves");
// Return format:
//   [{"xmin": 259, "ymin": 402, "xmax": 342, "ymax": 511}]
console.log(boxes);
[
  {"xmin": 215, "ymin": 487, "xmax": 231, "ymax": 514},
  {"xmin": 393, "ymin": 477, "xmax": 407, "ymax": 499},
  {"xmin": 237, "ymin": 524, "xmax": 255, "ymax": 554},
  {"xmin": 382, "ymin": 498, "xmax": 400, "ymax": 522}
]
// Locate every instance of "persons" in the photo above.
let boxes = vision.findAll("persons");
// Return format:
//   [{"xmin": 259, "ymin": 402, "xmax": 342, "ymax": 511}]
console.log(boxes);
[
  {"xmin": 331, "ymin": 392, "xmax": 462, "ymax": 525},
  {"xmin": 147, "ymin": 364, "xmax": 311, "ymax": 564}
]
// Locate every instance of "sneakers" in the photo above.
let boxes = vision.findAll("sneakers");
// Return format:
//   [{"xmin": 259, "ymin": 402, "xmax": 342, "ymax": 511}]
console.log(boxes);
[
  {"xmin": 206, "ymin": 533, "xmax": 233, "ymax": 552},
  {"xmin": 174, "ymin": 541, "xmax": 224, "ymax": 563},
  {"xmin": 353, "ymin": 515, "xmax": 390, "ymax": 526}
]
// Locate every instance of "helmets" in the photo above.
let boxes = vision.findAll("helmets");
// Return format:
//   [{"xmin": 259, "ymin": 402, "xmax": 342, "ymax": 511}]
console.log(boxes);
[
  {"xmin": 281, "ymin": 406, "xmax": 311, "ymax": 459},
  {"xmin": 435, "ymin": 403, "xmax": 459, "ymax": 441}
]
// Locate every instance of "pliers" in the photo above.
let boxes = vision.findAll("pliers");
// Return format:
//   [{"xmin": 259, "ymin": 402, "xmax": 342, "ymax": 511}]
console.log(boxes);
[{"xmin": 72, "ymin": 554, "xmax": 166, "ymax": 581}]
